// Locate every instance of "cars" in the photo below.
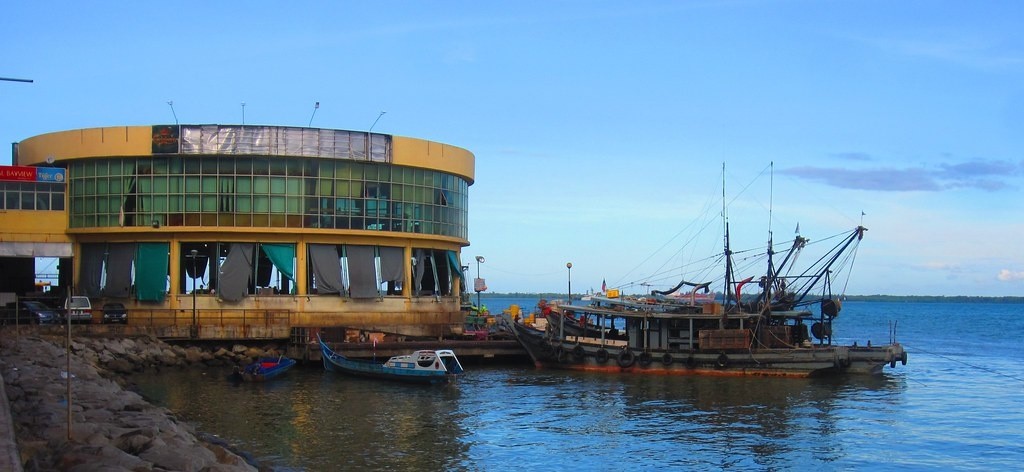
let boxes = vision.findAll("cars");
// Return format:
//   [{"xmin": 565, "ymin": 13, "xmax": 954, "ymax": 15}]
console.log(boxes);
[{"xmin": 100, "ymin": 302, "xmax": 129, "ymax": 325}]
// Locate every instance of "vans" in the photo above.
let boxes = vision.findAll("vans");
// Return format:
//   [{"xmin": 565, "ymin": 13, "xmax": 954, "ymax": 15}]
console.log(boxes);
[
  {"xmin": 56, "ymin": 295, "xmax": 92, "ymax": 323},
  {"xmin": 6, "ymin": 301, "xmax": 54, "ymax": 324}
]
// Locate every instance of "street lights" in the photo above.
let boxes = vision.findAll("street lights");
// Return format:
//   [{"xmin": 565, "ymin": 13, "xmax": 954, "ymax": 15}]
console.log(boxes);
[
  {"xmin": 475, "ymin": 256, "xmax": 485, "ymax": 317},
  {"xmin": 191, "ymin": 249, "xmax": 198, "ymax": 327},
  {"xmin": 566, "ymin": 262, "xmax": 572, "ymax": 306}
]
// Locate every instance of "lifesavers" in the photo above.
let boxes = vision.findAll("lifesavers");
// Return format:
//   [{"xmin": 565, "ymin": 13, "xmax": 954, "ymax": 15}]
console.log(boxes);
[
  {"xmin": 661, "ymin": 353, "xmax": 674, "ymax": 366},
  {"xmin": 638, "ymin": 352, "xmax": 652, "ymax": 367},
  {"xmin": 595, "ymin": 349, "xmax": 610, "ymax": 363},
  {"xmin": 616, "ymin": 350, "xmax": 635, "ymax": 368},
  {"xmin": 572, "ymin": 347, "xmax": 585, "ymax": 359},
  {"xmin": 557, "ymin": 345, "xmax": 567, "ymax": 358},
  {"xmin": 716, "ymin": 355, "xmax": 729, "ymax": 367},
  {"xmin": 685, "ymin": 357, "xmax": 697, "ymax": 369}
]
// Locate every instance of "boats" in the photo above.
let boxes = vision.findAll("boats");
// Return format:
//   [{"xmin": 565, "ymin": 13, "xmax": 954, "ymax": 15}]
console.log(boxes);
[
  {"xmin": 314, "ymin": 331, "xmax": 466, "ymax": 382},
  {"xmin": 237, "ymin": 356, "xmax": 297, "ymax": 384},
  {"xmin": 499, "ymin": 158, "xmax": 909, "ymax": 379}
]
